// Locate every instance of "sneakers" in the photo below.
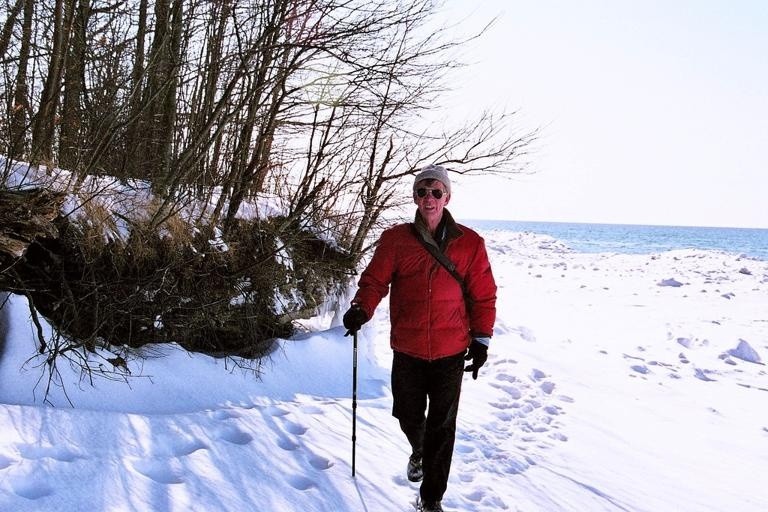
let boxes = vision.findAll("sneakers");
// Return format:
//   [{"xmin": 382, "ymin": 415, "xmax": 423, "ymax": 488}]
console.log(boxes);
[
  {"xmin": 418, "ymin": 492, "xmax": 443, "ymax": 512},
  {"xmin": 406, "ymin": 446, "xmax": 423, "ymax": 483}
]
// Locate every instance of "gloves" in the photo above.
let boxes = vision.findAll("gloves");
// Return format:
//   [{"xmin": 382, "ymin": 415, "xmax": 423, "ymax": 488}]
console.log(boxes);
[
  {"xmin": 342, "ymin": 307, "xmax": 367, "ymax": 337},
  {"xmin": 464, "ymin": 339, "xmax": 488, "ymax": 380}
]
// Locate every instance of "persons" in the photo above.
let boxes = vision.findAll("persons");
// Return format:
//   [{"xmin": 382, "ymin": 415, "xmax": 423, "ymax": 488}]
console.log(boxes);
[{"xmin": 343, "ymin": 164, "xmax": 497, "ymax": 512}]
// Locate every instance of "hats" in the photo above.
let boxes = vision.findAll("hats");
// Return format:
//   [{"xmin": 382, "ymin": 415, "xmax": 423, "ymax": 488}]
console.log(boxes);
[{"xmin": 413, "ymin": 165, "xmax": 451, "ymax": 192}]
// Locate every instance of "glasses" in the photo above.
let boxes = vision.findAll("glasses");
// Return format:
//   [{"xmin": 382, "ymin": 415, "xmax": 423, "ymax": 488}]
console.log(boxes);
[{"xmin": 416, "ymin": 187, "xmax": 447, "ymax": 199}]
